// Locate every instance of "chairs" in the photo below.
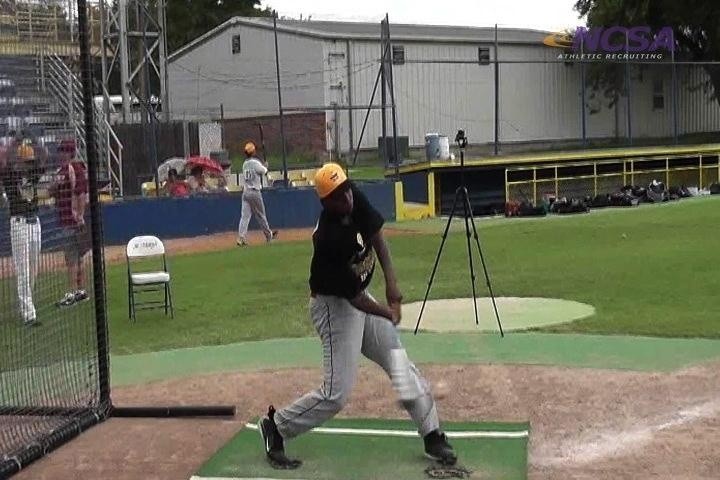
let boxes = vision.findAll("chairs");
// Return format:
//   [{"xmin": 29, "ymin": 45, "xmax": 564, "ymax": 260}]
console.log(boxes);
[
  {"xmin": 126, "ymin": 235, "xmax": 174, "ymax": 324},
  {"xmin": 97, "ymin": 194, "xmax": 111, "ymax": 201},
  {"xmin": 141, "ymin": 168, "xmax": 320, "ymax": 196}
]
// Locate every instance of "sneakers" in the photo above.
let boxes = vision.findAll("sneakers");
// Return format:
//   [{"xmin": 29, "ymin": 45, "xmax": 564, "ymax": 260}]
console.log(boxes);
[
  {"xmin": 258, "ymin": 405, "xmax": 302, "ymax": 469},
  {"xmin": 56, "ymin": 291, "xmax": 90, "ymax": 308},
  {"xmin": 25, "ymin": 320, "xmax": 42, "ymax": 327},
  {"xmin": 423, "ymin": 428, "xmax": 457, "ymax": 465}
]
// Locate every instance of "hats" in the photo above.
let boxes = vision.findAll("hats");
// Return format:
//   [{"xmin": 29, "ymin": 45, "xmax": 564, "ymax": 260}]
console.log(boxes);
[
  {"xmin": 57, "ymin": 139, "xmax": 76, "ymax": 153},
  {"xmin": 314, "ymin": 163, "xmax": 347, "ymax": 197},
  {"xmin": 17, "ymin": 145, "xmax": 34, "ymax": 161},
  {"xmin": 244, "ymin": 142, "xmax": 255, "ymax": 154}
]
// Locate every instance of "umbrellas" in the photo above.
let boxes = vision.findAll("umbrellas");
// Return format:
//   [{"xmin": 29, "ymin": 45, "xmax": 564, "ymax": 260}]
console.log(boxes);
[
  {"xmin": 158, "ymin": 156, "xmax": 187, "ymax": 182},
  {"xmin": 186, "ymin": 155, "xmax": 223, "ymax": 174}
]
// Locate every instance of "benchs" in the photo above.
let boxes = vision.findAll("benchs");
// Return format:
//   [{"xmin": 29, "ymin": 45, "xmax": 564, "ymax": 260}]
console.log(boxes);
[{"xmin": 0, "ymin": 64, "xmax": 94, "ymax": 215}]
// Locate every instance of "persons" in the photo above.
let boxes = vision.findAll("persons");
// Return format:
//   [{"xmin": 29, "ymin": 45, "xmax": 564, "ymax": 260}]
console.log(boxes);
[
  {"xmin": 234, "ymin": 141, "xmax": 278, "ymax": 245},
  {"xmin": 165, "ymin": 166, "xmax": 225, "ymax": 196},
  {"xmin": 258, "ymin": 162, "xmax": 458, "ymax": 468},
  {"xmin": 1, "ymin": 130, "xmax": 34, "ymax": 169},
  {"xmin": 38, "ymin": 139, "xmax": 85, "ymax": 309},
  {"xmin": 3, "ymin": 144, "xmax": 45, "ymax": 328}
]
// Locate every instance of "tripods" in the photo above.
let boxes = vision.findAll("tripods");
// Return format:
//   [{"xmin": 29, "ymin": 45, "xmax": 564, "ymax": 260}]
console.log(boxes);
[{"xmin": 415, "ymin": 129, "xmax": 504, "ymax": 337}]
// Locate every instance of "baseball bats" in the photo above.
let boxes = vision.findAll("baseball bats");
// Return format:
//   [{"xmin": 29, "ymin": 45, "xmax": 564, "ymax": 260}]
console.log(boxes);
[{"xmin": 391, "ymin": 301, "xmax": 418, "ymax": 410}]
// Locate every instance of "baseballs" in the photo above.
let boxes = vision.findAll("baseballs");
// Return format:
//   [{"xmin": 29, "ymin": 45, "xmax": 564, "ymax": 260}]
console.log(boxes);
[{"xmin": 432, "ymin": 381, "xmax": 448, "ymax": 400}]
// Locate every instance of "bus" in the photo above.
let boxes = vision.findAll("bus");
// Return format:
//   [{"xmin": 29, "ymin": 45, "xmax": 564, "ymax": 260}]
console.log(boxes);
[{"xmin": 94, "ymin": 93, "xmax": 163, "ymax": 124}]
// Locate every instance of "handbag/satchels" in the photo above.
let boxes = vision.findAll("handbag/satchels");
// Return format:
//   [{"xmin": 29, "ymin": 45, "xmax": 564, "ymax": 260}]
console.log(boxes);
[{"xmin": 504, "ymin": 180, "xmax": 719, "ymax": 217}]
